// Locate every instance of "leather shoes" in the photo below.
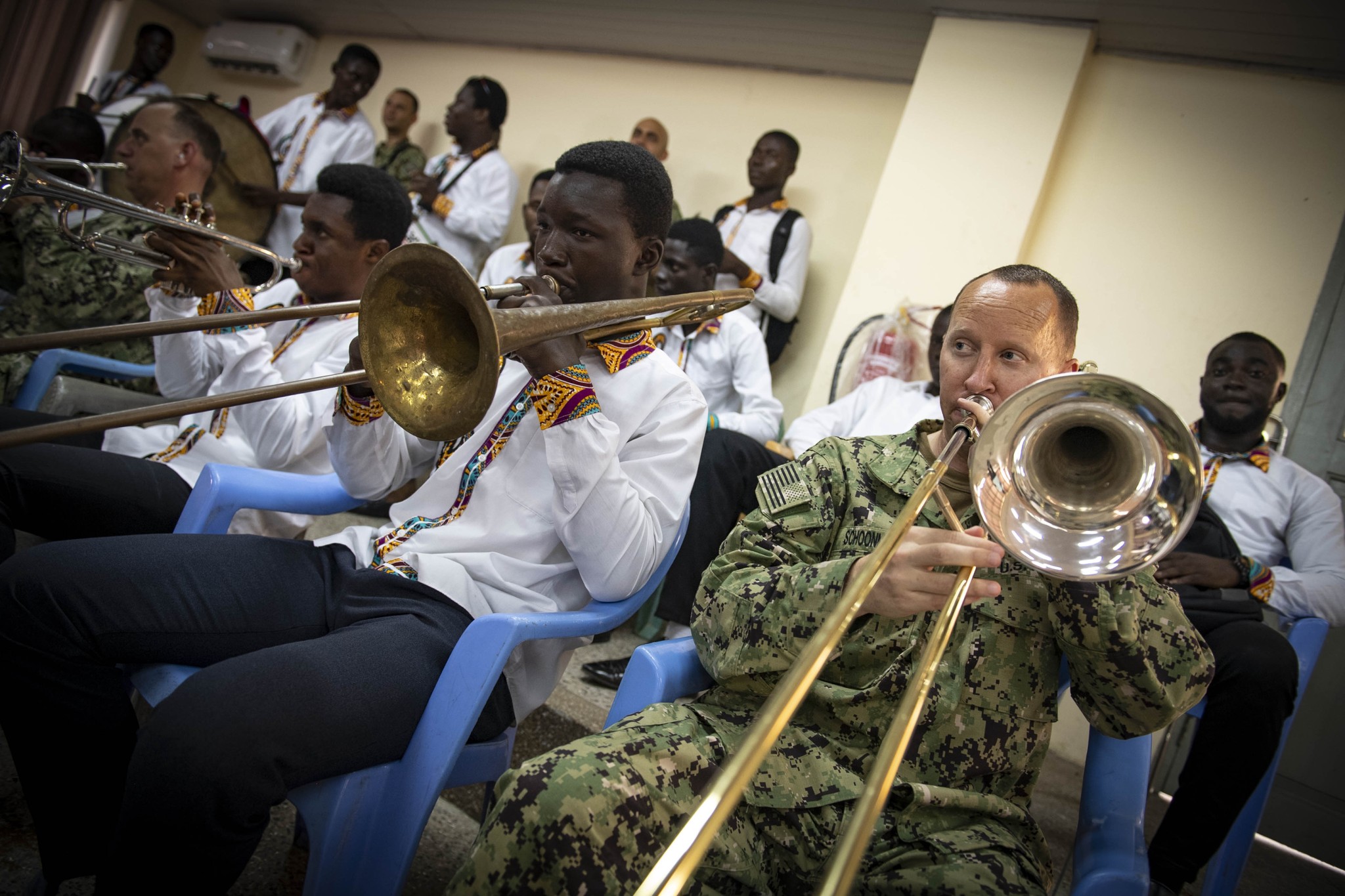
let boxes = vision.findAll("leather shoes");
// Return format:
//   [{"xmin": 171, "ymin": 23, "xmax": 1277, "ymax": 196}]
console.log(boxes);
[{"xmin": 580, "ymin": 633, "xmax": 668, "ymax": 692}]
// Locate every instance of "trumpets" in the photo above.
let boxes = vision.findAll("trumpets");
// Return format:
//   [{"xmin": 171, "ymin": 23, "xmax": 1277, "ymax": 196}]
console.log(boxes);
[
  {"xmin": 0, "ymin": 130, "xmax": 303, "ymax": 295},
  {"xmin": 35, "ymin": 162, "xmax": 128, "ymax": 170}
]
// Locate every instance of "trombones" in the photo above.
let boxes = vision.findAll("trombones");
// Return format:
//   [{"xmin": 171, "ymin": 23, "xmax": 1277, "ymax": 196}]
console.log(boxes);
[
  {"xmin": 0, "ymin": 243, "xmax": 755, "ymax": 448},
  {"xmin": 633, "ymin": 362, "xmax": 1203, "ymax": 896}
]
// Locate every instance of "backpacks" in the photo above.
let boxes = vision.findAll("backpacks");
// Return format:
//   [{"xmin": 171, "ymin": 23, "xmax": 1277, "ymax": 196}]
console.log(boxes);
[
  {"xmin": 1176, "ymin": 497, "xmax": 1264, "ymax": 630},
  {"xmin": 715, "ymin": 204, "xmax": 801, "ymax": 363}
]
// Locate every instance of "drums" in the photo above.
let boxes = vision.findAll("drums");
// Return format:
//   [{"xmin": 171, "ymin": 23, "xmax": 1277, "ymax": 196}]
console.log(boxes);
[{"xmin": 94, "ymin": 94, "xmax": 279, "ymax": 267}]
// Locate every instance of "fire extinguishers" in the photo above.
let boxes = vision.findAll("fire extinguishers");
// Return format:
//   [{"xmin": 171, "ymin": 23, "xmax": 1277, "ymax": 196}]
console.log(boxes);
[{"xmin": 828, "ymin": 302, "xmax": 943, "ymax": 403}]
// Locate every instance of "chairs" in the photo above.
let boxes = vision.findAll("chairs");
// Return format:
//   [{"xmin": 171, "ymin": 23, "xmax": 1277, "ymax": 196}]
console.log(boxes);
[
  {"xmin": 18, "ymin": 347, "xmax": 157, "ymax": 411},
  {"xmin": 605, "ymin": 611, "xmax": 1156, "ymax": 896},
  {"xmin": 39, "ymin": 376, "xmax": 179, "ymax": 429},
  {"xmin": 1060, "ymin": 605, "xmax": 1331, "ymax": 896},
  {"xmin": 121, "ymin": 460, "xmax": 693, "ymax": 896}
]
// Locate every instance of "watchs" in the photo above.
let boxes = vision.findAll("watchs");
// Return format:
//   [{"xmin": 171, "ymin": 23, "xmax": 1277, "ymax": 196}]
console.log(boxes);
[{"xmin": 1235, "ymin": 555, "xmax": 1250, "ymax": 588}]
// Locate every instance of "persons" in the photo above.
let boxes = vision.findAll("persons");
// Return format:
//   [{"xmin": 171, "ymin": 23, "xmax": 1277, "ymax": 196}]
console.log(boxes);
[
  {"xmin": 0, "ymin": 100, "xmax": 711, "ymax": 895},
  {"xmin": 1148, "ymin": 332, "xmax": 1345, "ymax": 896},
  {"xmin": 581, "ymin": 304, "xmax": 954, "ymax": 690},
  {"xmin": 54, "ymin": 23, "xmax": 813, "ymax": 449},
  {"xmin": 447, "ymin": 264, "xmax": 1214, "ymax": 896}
]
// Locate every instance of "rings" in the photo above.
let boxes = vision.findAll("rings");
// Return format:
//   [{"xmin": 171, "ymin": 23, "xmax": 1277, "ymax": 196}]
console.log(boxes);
[{"xmin": 144, "ymin": 231, "xmax": 158, "ymax": 250}]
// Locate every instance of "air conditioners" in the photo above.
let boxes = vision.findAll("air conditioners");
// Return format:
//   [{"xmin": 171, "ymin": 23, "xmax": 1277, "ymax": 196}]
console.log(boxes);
[{"xmin": 197, "ymin": 19, "xmax": 316, "ymax": 85}]
